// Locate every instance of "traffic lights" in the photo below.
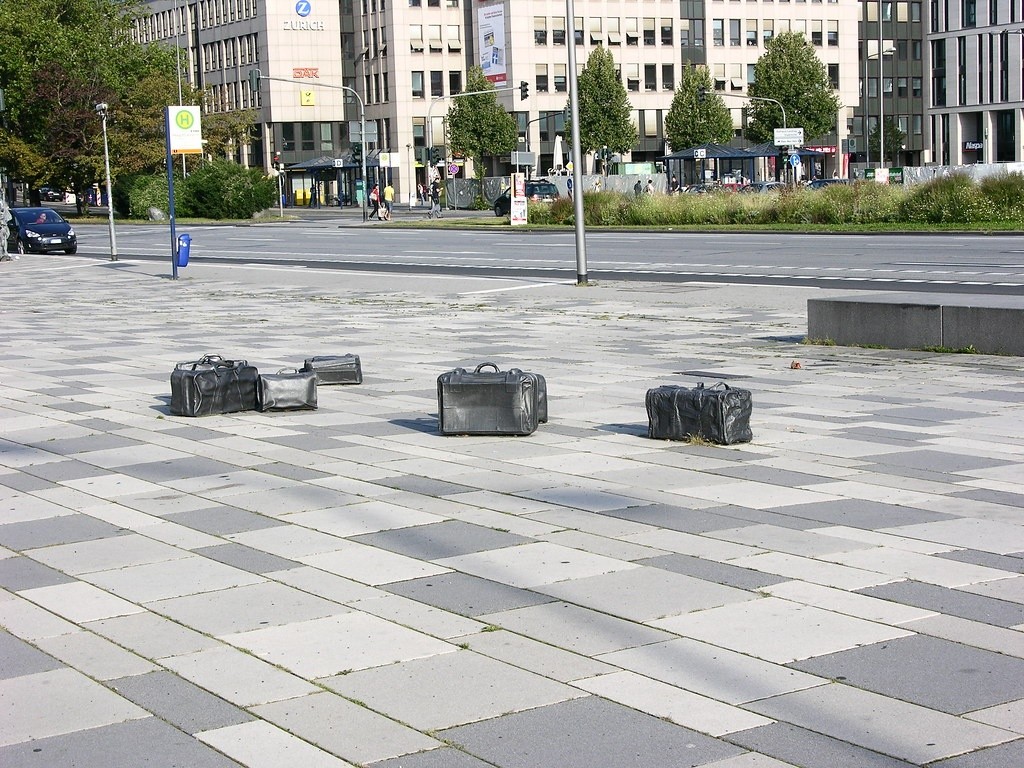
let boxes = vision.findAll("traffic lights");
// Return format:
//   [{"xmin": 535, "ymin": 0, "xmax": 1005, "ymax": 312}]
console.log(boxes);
[
  {"xmin": 698, "ymin": 86, "xmax": 706, "ymax": 103},
  {"xmin": 521, "ymin": 81, "xmax": 529, "ymax": 101}
]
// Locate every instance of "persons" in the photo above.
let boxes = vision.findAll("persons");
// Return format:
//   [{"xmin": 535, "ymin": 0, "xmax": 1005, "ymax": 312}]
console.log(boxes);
[
  {"xmin": 308, "ymin": 185, "xmax": 317, "ymax": 207},
  {"xmin": 418, "ymin": 184, "xmax": 425, "ymax": 206},
  {"xmin": 669, "ymin": 176, "xmax": 680, "ymax": 196},
  {"xmin": 427, "ymin": 177, "xmax": 444, "ymax": 219},
  {"xmin": 369, "ymin": 184, "xmax": 383, "ymax": 221},
  {"xmin": 643, "ymin": 179, "xmax": 655, "ymax": 197},
  {"xmin": 36, "ymin": 213, "xmax": 50, "ymax": 224},
  {"xmin": 633, "ymin": 180, "xmax": 642, "ymax": 198},
  {"xmin": 382, "ymin": 181, "xmax": 395, "ymax": 221}
]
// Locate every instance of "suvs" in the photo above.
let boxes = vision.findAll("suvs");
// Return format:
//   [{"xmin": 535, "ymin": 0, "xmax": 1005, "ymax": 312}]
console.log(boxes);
[
  {"xmin": 38, "ymin": 187, "xmax": 63, "ymax": 201},
  {"xmin": 494, "ymin": 178, "xmax": 562, "ymax": 216}
]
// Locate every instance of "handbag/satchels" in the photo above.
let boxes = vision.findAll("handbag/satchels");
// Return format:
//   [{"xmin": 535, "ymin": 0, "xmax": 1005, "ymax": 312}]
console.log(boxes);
[
  {"xmin": 673, "ymin": 191, "xmax": 679, "ymax": 197},
  {"xmin": 369, "ymin": 188, "xmax": 377, "ymax": 200},
  {"xmin": 433, "ymin": 199, "xmax": 439, "ymax": 204},
  {"xmin": 378, "ymin": 206, "xmax": 386, "ymax": 217},
  {"xmin": 644, "ymin": 382, "xmax": 753, "ymax": 444},
  {"xmin": 437, "ymin": 363, "xmax": 548, "ymax": 434},
  {"xmin": 257, "ymin": 367, "xmax": 319, "ymax": 410},
  {"xmin": 171, "ymin": 353, "xmax": 258, "ymax": 416},
  {"xmin": 302, "ymin": 354, "xmax": 361, "ymax": 384},
  {"xmin": 643, "ymin": 185, "xmax": 649, "ymax": 193}
]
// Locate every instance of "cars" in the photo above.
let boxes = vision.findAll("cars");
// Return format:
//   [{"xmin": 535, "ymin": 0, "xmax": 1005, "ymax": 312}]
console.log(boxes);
[
  {"xmin": 685, "ymin": 178, "xmax": 848, "ymax": 196},
  {"xmin": 6, "ymin": 206, "xmax": 77, "ymax": 254}
]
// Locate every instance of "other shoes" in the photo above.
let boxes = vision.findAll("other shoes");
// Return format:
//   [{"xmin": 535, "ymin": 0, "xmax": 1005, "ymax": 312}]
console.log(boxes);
[
  {"xmin": 426, "ymin": 213, "xmax": 432, "ymax": 219},
  {"xmin": 437, "ymin": 216, "xmax": 444, "ymax": 218}
]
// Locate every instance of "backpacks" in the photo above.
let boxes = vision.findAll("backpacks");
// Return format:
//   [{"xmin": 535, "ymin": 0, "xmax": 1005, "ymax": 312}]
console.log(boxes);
[
  {"xmin": 428, "ymin": 184, "xmax": 434, "ymax": 196},
  {"xmin": 424, "ymin": 186, "xmax": 426, "ymax": 192}
]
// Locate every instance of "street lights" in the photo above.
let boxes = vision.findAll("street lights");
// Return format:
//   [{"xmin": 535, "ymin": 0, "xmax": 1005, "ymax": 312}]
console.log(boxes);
[{"xmin": 865, "ymin": 47, "xmax": 896, "ymax": 169}]
